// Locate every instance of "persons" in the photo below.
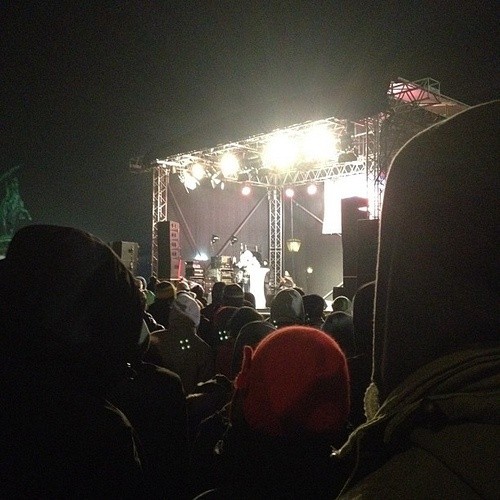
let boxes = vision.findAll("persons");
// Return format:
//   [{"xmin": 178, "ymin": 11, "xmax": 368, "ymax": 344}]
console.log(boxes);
[
  {"xmin": 193, "ymin": 325, "xmax": 350, "ymax": 500},
  {"xmin": 331, "ymin": 98, "xmax": 499, "ymax": 500},
  {"xmin": 1, "ymin": 224, "xmax": 143, "ymax": 500},
  {"xmin": 236, "ymin": 250, "xmax": 260, "ymax": 292},
  {"xmin": 135, "ymin": 275, "xmax": 377, "ymax": 500}
]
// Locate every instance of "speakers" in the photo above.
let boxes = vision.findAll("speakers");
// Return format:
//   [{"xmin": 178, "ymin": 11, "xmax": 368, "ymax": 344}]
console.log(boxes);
[
  {"xmin": 157, "ymin": 221, "xmax": 180, "ymax": 290},
  {"xmin": 333, "ymin": 197, "xmax": 380, "ymax": 300},
  {"xmin": 113, "ymin": 241, "xmax": 138, "ymax": 278}
]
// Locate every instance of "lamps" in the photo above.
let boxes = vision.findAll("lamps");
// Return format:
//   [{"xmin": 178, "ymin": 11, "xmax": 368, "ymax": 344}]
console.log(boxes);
[{"xmin": 285, "ymin": 238, "xmax": 302, "ymax": 252}]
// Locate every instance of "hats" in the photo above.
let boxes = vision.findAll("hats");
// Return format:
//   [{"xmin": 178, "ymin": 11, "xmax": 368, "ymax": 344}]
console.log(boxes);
[
  {"xmin": 234, "ymin": 325, "xmax": 349, "ymax": 438},
  {"xmin": 156, "ymin": 281, "xmax": 175, "ymax": 304},
  {"xmin": 331, "ymin": 296, "xmax": 352, "ymax": 313}
]
